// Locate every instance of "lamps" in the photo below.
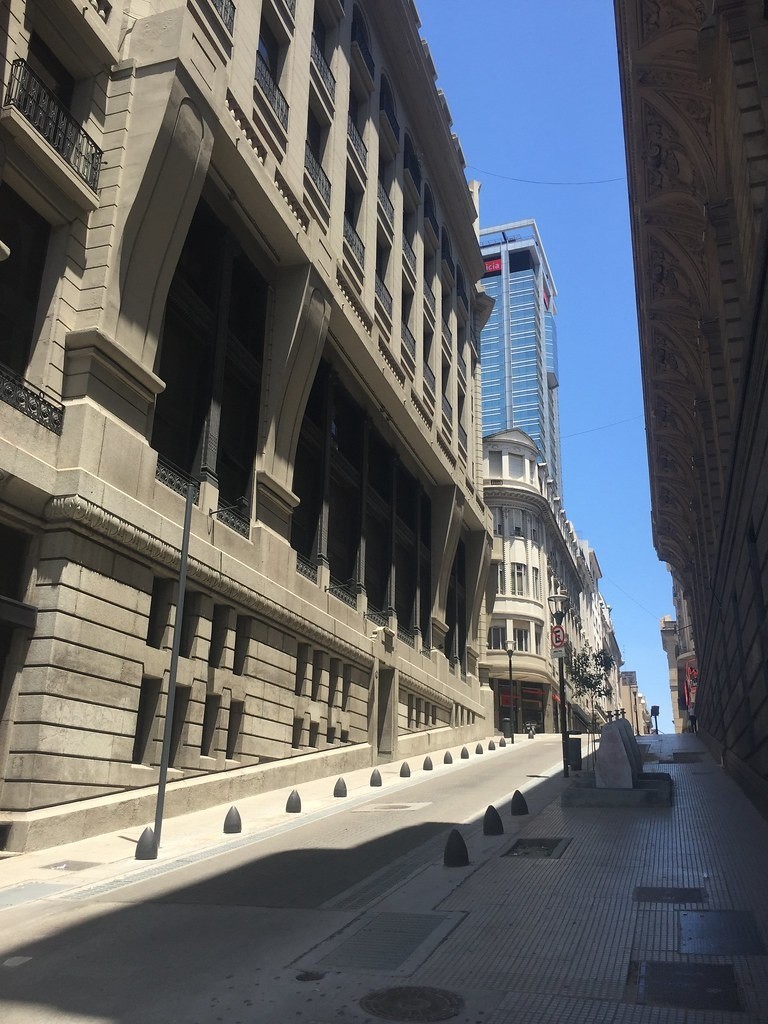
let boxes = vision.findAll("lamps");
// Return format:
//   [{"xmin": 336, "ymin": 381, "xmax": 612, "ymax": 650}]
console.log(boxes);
[
  {"xmin": 209, "ymin": 496, "xmax": 249, "ymax": 517},
  {"xmin": 364, "ymin": 607, "xmax": 444, "ymax": 654},
  {"xmin": 325, "ymin": 578, "xmax": 357, "ymax": 593}
]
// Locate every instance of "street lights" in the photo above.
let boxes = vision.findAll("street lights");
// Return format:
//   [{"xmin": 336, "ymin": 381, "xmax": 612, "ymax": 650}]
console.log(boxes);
[
  {"xmin": 546, "ymin": 595, "xmax": 572, "ymax": 778},
  {"xmin": 504, "ymin": 639, "xmax": 516, "ymax": 744},
  {"xmin": 633, "ymin": 688, "xmax": 642, "ymax": 737}
]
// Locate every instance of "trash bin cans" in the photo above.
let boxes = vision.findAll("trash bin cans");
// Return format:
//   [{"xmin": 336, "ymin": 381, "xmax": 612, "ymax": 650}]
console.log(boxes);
[
  {"xmin": 502, "ymin": 717, "xmax": 512, "ymax": 738},
  {"xmin": 565, "ymin": 729, "xmax": 583, "ymax": 771}
]
